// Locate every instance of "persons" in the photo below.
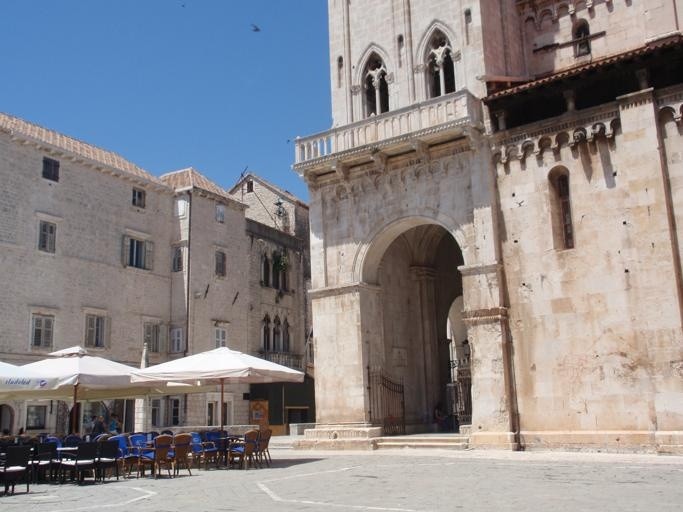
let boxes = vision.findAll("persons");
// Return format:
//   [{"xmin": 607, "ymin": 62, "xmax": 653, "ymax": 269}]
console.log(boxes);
[{"xmin": 433, "ymin": 401, "xmax": 448, "ymax": 433}]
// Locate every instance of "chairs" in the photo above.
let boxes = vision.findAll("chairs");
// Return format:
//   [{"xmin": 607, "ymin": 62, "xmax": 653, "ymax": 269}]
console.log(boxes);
[{"xmin": 0, "ymin": 424, "xmax": 279, "ymax": 499}]
